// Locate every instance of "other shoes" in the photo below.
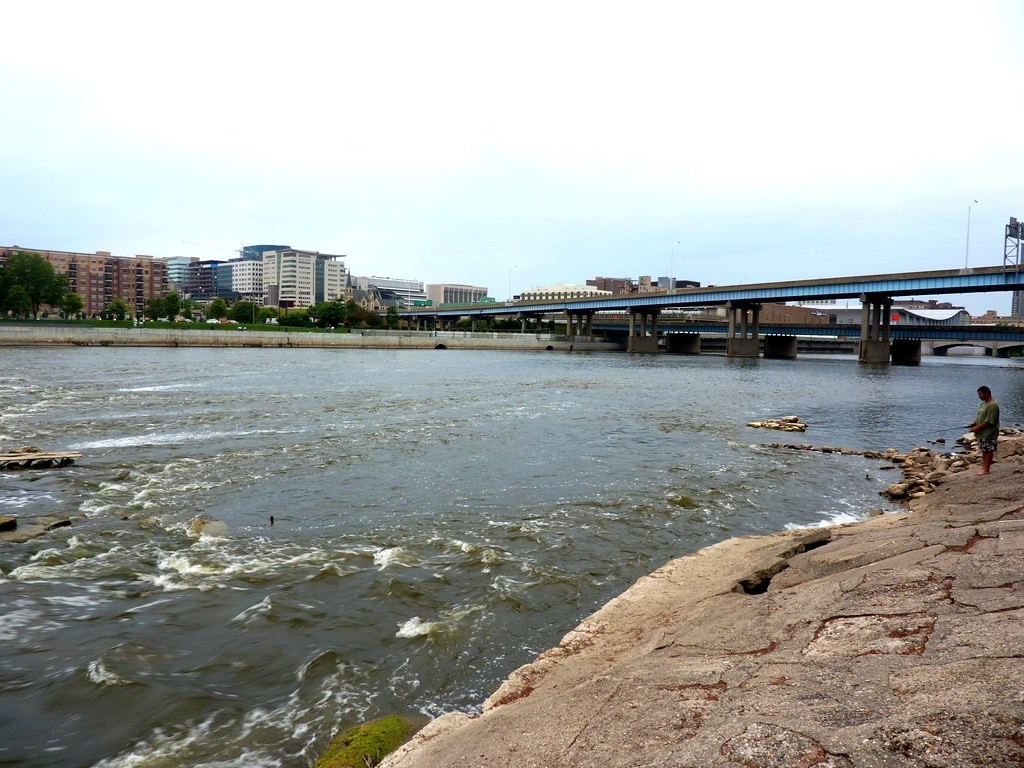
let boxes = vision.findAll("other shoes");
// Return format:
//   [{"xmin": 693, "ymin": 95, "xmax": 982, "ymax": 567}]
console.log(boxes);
[
  {"xmin": 978, "ymin": 471, "xmax": 983, "ymax": 472},
  {"xmin": 978, "ymin": 471, "xmax": 990, "ymax": 475}
]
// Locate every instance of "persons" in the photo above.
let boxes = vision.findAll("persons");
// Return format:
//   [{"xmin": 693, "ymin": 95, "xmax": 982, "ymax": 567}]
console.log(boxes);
[{"xmin": 968, "ymin": 385, "xmax": 1000, "ymax": 476}]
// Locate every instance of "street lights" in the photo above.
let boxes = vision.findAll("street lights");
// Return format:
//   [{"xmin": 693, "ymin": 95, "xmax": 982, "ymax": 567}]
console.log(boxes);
[
  {"xmin": 670, "ymin": 241, "xmax": 681, "ymax": 290},
  {"xmin": 479, "ymin": 311, "xmax": 483, "ymax": 332},
  {"xmin": 964, "ymin": 200, "xmax": 978, "ymax": 269},
  {"xmin": 143, "ymin": 298, "xmax": 146, "ymax": 322},
  {"xmin": 509, "ymin": 265, "xmax": 518, "ymax": 306},
  {"xmin": 409, "ymin": 280, "xmax": 418, "ymax": 308}
]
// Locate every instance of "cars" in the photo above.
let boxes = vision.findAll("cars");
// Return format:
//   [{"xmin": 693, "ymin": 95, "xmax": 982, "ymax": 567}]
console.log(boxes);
[
  {"xmin": 10, "ymin": 313, "xmax": 19, "ymax": 321},
  {"xmin": 157, "ymin": 318, "xmax": 170, "ymax": 322},
  {"xmin": 224, "ymin": 320, "xmax": 239, "ymax": 324},
  {"xmin": 206, "ymin": 319, "xmax": 221, "ymax": 324},
  {"xmin": 176, "ymin": 319, "xmax": 194, "ymax": 323}
]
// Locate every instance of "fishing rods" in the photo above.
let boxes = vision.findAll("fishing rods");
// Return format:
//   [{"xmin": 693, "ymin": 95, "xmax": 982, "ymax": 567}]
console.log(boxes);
[{"xmin": 897, "ymin": 425, "xmax": 978, "ymax": 442}]
